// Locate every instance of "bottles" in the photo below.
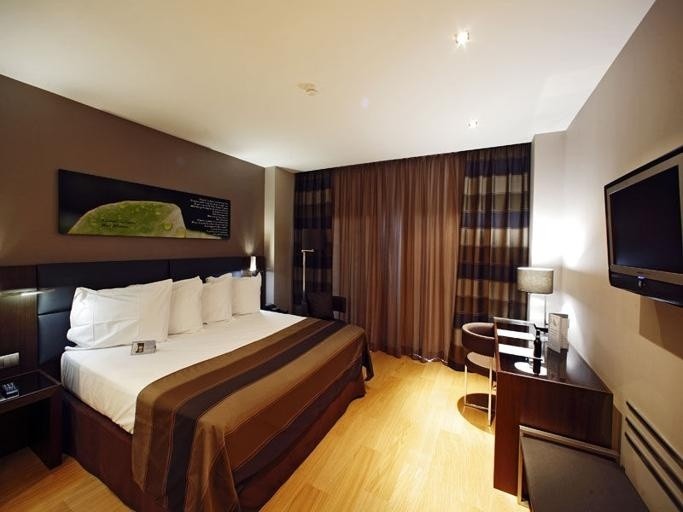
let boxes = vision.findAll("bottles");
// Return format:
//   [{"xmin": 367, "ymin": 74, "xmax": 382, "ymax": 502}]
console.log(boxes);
[
  {"xmin": 533, "ymin": 359, "xmax": 540, "ymax": 376},
  {"xmin": 534, "ymin": 330, "xmax": 541, "ymax": 358}
]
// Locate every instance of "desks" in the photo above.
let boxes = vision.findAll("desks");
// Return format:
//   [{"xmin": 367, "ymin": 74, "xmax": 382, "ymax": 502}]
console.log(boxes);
[
  {"xmin": 492, "ymin": 315, "xmax": 614, "ymax": 497},
  {"xmin": 517, "ymin": 424, "xmax": 651, "ymax": 512}
]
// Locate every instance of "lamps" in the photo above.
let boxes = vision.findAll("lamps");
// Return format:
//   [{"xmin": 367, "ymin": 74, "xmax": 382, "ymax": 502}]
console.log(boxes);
[
  {"xmin": 300, "ymin": 228, "xmax": 324, "ymax": 313},
  {"xmin": 517, "ymin": 267, "xmax": 554, "ymax": 331},
  {"xmin": 250, "ymin": 256, "xmax": 257, "ymax": 272}
]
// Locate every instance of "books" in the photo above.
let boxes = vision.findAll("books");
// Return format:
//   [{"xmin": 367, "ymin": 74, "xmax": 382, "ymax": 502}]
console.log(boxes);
[{"xmin": 547, "ymin": 313, "xmax": 569, "ymax": 354}]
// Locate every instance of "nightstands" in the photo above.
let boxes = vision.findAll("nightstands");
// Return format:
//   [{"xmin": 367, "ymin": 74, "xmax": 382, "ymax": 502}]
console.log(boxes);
[{"xmin": 0, "ymin": 368, "xmax": 63, "ymax": 470}]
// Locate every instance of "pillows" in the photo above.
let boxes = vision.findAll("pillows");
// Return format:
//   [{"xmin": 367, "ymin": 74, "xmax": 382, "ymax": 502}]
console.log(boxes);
[
  {"xmin": 201, "ymin": 278, "xmax": 232, "ymax": 324},
  {"xmin": 231, "ymin": 272, "xmax": 262, "ymax": 315},
  {"xmin": 168, "ymin": 276, "xmax": 203, "ymax": 335},
  {"xmin": 66, "ymin": 278, "xmax": 172, "ymax": 351}
]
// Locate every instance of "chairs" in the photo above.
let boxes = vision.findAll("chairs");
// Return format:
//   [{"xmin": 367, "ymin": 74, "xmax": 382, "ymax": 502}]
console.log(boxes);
[{"xmin": 461, "ymin": 322, "xmax": 496, "ymax": 426}]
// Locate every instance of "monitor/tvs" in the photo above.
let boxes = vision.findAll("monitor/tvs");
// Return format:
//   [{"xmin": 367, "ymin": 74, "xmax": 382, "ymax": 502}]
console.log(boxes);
[{"xmin": 604, "ymin": 145, "xmax": 683, "ymax": 307}]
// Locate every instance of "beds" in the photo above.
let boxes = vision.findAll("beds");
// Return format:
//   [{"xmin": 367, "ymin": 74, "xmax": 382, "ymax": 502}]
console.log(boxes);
[{"xmin": 38, "ymin": 257, "xmax": 365, "ymax": 512}]
